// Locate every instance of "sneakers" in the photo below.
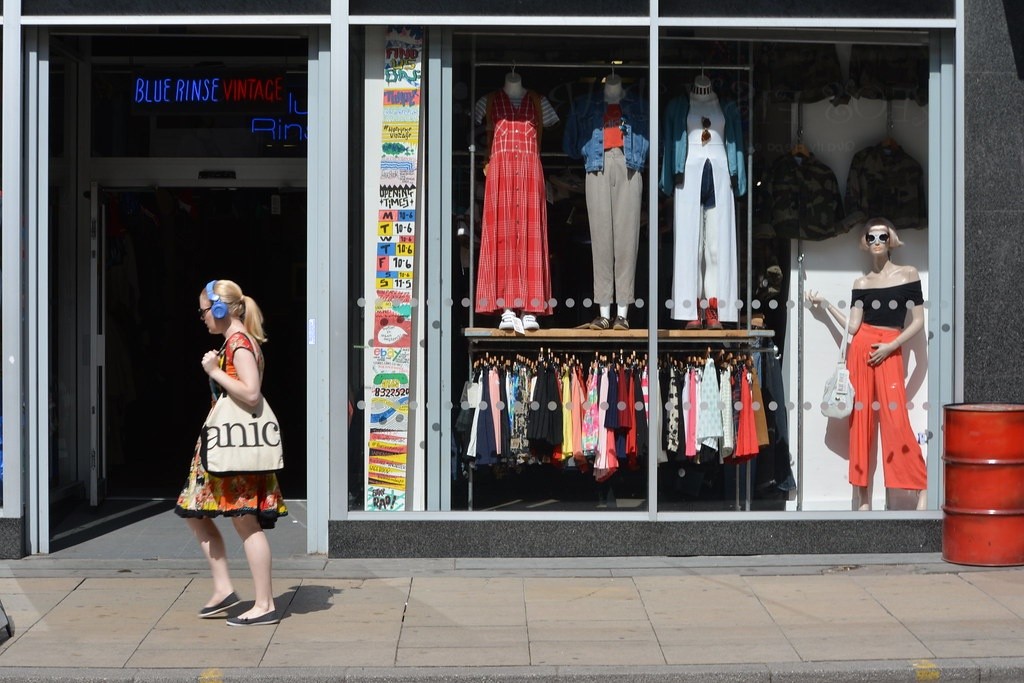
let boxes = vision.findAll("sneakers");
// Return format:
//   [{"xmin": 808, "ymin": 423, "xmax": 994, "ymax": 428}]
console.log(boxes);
[
  {"xmin": 590, "ymin": 317, "xmax": 614, "ymax": 330},
  {"xmin": 519, "ymin": 312, "xmax": 539, "ymax": 329},
  {"xmin": 613, "ymin": 316, "xmax": 629, "ymax": 329},
  {"xmin": 499, "ymin": 312, "xmax": 516, "ymax": 330}
]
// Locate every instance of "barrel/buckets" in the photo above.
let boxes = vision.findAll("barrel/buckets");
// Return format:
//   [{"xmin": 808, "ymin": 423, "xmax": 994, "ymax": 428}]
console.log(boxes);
[{"xmin": 942, "ymin": 402, "xmax": 1024, "ymax": 566}]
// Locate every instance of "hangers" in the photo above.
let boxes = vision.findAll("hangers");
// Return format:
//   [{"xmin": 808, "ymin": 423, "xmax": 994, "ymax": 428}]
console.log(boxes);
[
  {"xmin": 880, "ymin": 121, "xmax": 900, "ymax": 153},
  {"xmin": 790, "ymin": 128, "xmax": 810, "ymax": 158},
  {"xmin": 471, "ymin": 349, "xmax": 755, "ymax": 375}
]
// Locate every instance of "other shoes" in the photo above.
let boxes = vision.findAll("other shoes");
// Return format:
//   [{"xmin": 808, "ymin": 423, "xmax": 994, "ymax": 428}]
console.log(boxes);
[
  {"xmin": 198, "ymin": 592, "xmax": 241, "ymax": 617},
  {"xmin": 226, "ymin": 610, "xmax": 279, "ymax": 626}
]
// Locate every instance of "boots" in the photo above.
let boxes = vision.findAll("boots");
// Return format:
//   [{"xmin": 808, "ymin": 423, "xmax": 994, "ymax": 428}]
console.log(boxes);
[
  {"xmin": 705, "ymin": 298, "xmax": 723, "ymax": 329},
  {"xmin": 685, "ymin": 298, "xmax": 702, "ymax": 330}
]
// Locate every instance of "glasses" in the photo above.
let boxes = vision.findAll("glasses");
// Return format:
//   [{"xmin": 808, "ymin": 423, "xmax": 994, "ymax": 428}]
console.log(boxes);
[
  {"xmin": 864, "ymin": 233, "xmax": 889, "ymax": 243},
  {"xmin": 198, "ymin": 305, "xmax": 213, "ymax": 318},
  {"xmin": 701, "ymin": 117, "xmax": 711, "ymax": 145}
]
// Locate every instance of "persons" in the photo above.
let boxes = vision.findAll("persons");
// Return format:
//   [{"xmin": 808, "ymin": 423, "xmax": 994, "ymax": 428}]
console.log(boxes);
[
  {"xmin": 568, "ymin": 73, "xmax": 648, "ymax": 331},
  {"xmin": 174, "ymin": 279, "xmax": 290, "ymax": 626},
  {"xmin": 468, "ymin": 72, "xmax": 560, "ymax": 332},
  {"xmin": 809, "ymin": 215, "xmax": 928, "ymax": 510},
  {"xmin": 659, "ymin": 74, "xmax": 746, "ymax": 330}
]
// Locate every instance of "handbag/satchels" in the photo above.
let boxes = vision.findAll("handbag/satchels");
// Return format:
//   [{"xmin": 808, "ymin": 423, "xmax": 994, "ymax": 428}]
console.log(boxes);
[
  {"xmin": 200, "ymin": 331, "xmax": 285, "ymax": 477},
  {"xmin": 821, "ymin": 328, "xmax": 855, "ymax": 419}
]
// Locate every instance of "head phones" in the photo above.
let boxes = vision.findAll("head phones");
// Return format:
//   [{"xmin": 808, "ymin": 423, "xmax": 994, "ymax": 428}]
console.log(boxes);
[{"xmin": 205, "ymin": 280, "xmax": 228, "ymax": 319}]
